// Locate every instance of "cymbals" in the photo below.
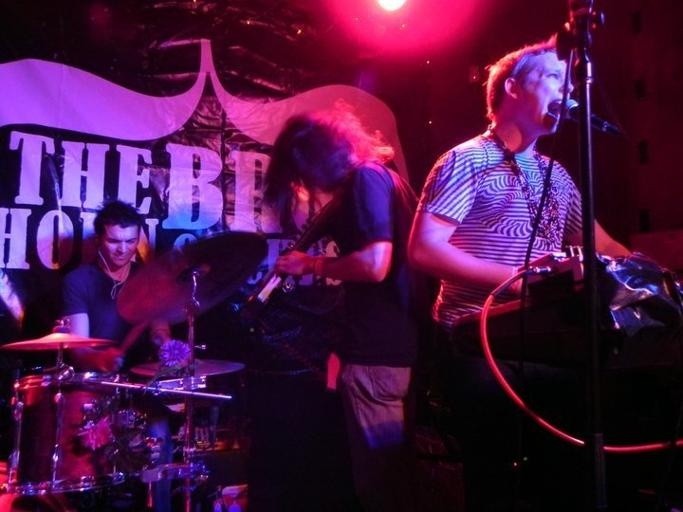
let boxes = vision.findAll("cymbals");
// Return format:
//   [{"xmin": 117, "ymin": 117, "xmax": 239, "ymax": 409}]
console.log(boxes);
[
  {"xmin": 116, "ymin": 233, "xmax": 270, "ymax": 326},
  {"xmin": 0, "ymin": 333, "xmax": 116, "ymax": 351},
  {"xmin": 129, "ymin": 357, "xmax": 245, "ymax": 379}
]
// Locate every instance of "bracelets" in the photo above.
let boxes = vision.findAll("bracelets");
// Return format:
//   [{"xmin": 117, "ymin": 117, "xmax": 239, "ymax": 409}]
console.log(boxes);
[
  {"xmin": 507, "ymin": 267, "xmax": 523, "ymax": 297},
  {"xmin": 312, "ymin": 254, "xmax": 328, "ymax": 277}
]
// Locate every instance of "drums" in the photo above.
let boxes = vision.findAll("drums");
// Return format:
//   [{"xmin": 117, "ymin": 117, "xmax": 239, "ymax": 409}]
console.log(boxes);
[
  {"xmin": 116, "ymin": 373, "xmax": 241, "ymax": 456},
  {"xmin": 9, "ymin": 371, "xmax": 128, "ymax": 496}
]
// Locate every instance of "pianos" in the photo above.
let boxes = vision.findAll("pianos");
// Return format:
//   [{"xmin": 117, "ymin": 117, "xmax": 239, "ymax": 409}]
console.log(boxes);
[{"xmin": 450, "ymin": 245, "xmax": 682, "ymax": 372}]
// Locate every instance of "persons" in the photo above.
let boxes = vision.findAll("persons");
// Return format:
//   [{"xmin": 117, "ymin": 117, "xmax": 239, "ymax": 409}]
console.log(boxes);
[
  {"xmin": 408, "ymin": 43, "xmax": 641, "ymax": 511},
  {"xmin": 261, "ymin": 115, "xmax": 436, "ymax": 509},
  {"xmin": 59, "ymin": 198, "xmax": 175, "ymax": 510}
]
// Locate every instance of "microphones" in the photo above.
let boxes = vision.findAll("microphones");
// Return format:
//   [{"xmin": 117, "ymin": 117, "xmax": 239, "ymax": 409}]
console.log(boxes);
[{"xmin": 561, "ymin": 98, "xmax": 621, "ymax": 137}]
